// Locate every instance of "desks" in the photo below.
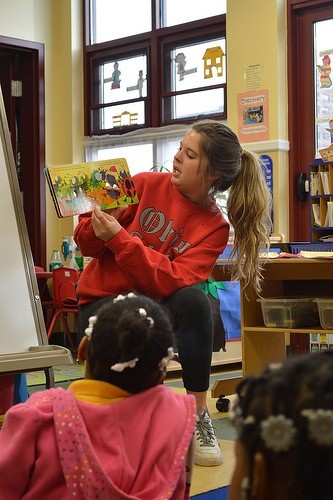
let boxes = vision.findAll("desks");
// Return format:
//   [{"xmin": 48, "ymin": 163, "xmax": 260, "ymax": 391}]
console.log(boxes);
[{"xmin": 37, "ymin": 271, "xmax": 81, "ymax": 279}]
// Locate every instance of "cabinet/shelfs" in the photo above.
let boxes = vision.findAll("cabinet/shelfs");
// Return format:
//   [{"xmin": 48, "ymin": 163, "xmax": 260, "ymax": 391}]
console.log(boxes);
[
  {"xmin": 210, "ymin": 255, "xmax": 333, "ymax": 414},
  {"xmin": 307, "ymin": 157, "xmax": 333, "ymax": 242}
]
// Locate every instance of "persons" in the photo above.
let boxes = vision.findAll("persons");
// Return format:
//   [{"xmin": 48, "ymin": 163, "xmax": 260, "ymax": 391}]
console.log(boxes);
[
  {"xmin": 0, "ymin": 291, "xmax": 193, "ymax": 499},
  {"xmin": 74, "ymin": 121, "xmax": 274, "ymax": 467},
  {"xmin": 228, "ymin": 344, "xmax": 333, "ymax": 500}
]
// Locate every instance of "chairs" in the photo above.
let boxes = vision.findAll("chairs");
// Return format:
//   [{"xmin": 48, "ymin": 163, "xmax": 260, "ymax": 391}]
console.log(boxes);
[{"xmin": 35, "ymin": 267, "xmax": 79, "ymax": 354}]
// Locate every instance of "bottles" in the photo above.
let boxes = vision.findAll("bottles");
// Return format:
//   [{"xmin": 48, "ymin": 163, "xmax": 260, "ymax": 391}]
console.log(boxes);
[
  {"xmin": 49, "ymin": 249, "xmax": 63, "ymax": 271},
  {"xmin": 60, "ymin": 236, "xmax": 71, "ymax": 268},
  {"xmin": 70, "ymin": 235, "xmax": 80, "ymax": 271},
  {"xmin": 74, "ymin": 247, "xmax": 83, "ymax": 274}
]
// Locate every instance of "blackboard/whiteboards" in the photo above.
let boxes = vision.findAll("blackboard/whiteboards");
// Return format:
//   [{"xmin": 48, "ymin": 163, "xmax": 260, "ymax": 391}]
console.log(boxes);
[{"xmin": 0, "ymin": 83, "xmax": 49, "ymax": 356}]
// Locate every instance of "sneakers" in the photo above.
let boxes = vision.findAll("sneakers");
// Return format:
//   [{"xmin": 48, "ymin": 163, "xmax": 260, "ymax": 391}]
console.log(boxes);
[{"xmin": 195, "ymin": 406, "xmax": 222, "ymax": 465}]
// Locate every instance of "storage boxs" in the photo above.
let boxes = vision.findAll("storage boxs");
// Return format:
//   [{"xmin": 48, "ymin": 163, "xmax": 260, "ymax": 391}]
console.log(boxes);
[
  {"xmin": 257, "ymin": 296, "xmax": 319, "ymax": 328},
  {"xmin": 312, "ymin": 297, "xmax": 333, "ymax": 330}
]
